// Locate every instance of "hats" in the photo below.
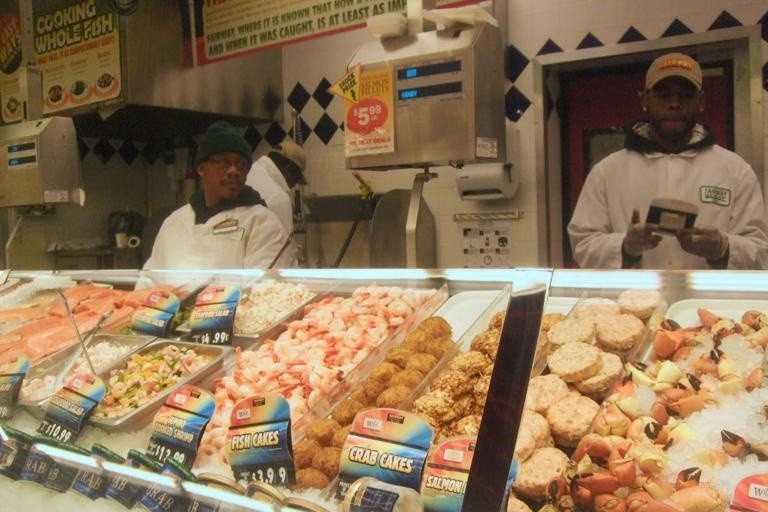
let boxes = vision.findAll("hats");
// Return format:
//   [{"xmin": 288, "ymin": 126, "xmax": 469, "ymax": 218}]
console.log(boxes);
[
  {"xmin": 646, "ymin": 52, "xmax": 703, "ymax": 92},
  {"xmin": 270, "ymin": 141, "xmax": 309, "ymax": 186},
  {"xmin": 192, "ymin": 119, "xmax": 252, "ymax": 172}
]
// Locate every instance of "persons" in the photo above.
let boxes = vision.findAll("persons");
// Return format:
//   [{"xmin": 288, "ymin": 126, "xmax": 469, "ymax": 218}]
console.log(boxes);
[
  {"xmin": 133, "ymin": 120, "xmax": 292, "ymax": 292},
  {"xmin": 244, "ymin": 137, "xmax": 309, "ymax": 242},
  {"xmin": 564, "ymin": 52, "xmax": 768, "ymax": 271}
]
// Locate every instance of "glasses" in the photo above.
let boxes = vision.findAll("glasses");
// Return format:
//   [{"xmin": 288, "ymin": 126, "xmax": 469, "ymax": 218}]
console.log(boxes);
[{"xmin": 204, "ymin": 157, "xmax": 251, "ymax": 170}]
